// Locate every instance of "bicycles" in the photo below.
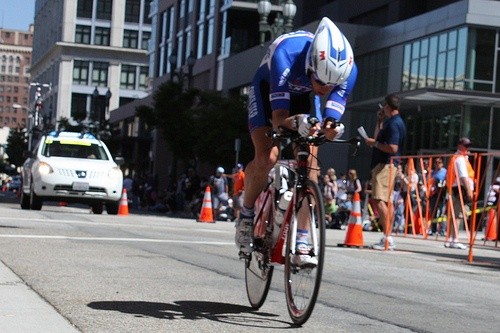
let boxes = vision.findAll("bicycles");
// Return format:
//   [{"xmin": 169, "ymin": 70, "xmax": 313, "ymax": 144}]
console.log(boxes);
[{"xmin": 243, "ymin": 116, "xmax": 360, "ymax": 325}]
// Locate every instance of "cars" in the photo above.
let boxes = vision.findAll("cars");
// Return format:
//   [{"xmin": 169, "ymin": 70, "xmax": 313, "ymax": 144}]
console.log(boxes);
[{"xmin": 20, "ymin": 131, "xmax": 124, "ymax": 215}]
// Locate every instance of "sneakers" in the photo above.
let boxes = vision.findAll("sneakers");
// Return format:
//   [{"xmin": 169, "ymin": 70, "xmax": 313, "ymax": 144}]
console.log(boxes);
[
  {"xmin": 233, "ymin": 216, "xmax": 254, "ymax": 254},
  {"xmin": 288, "ymin": 241, "xmax": 318, "ymax": 269}
]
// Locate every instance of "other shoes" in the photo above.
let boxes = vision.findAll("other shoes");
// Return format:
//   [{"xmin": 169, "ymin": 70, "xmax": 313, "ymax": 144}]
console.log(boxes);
[
  {"xmin": 444, "ymin": 242, "xmax": 467, "ymax": 250},
  {"xmin": 371, "ymin": 236, "xmax": 395, "ymax": 250}
]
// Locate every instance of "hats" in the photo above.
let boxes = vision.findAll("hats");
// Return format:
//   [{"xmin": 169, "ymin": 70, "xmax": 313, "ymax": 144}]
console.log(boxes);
[{"xmin": 216, "ymin": 167, "xmax": 225, "ymax": 174}]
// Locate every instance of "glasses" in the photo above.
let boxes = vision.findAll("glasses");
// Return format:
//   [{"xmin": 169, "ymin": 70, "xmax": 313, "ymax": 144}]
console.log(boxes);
[{"xmin": 313, "ymin": 74, "xmax": 326, "ymax": 86}]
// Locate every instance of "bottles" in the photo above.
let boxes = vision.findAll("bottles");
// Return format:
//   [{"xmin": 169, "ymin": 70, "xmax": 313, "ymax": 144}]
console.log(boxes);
[{"xmin": 274, "ymin": 191, "xmax": 293, "ymax": 229}]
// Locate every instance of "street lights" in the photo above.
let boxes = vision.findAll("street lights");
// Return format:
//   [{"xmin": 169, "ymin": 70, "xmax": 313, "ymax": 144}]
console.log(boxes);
[{"xmin": 11, "ymin": 104, "xmax": 38, "ymax": 147}]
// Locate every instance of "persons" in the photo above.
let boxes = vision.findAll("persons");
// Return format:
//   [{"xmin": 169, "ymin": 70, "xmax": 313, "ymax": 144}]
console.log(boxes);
[
  {"xmin": 444, "ymin": 137, "xmax": 474, "ymax": 249},
  {"xmin": 234, "ymin": 17, "xmax": 358, "ymax": 270},
  {"xmin": 365, "ymin": 93, "xmax": 408, "ymax": 251},
  {"xmin": 482, "ymin": 177, "xmax": 500, "ymax": 247},
  {"xmin": 123, "ymin": 158, "xmax": 447, "ymax": 232}
]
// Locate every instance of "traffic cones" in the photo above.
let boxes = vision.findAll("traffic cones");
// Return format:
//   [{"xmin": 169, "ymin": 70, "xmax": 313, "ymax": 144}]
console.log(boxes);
[
  {"xmin": 116, "ymin": 188, "xmax": 129, "ymax": 216},
  {"xmin": 197, "ymin": 186, "xmax": 214, "ymax": 222},
  {"xmin": 335, "ymin": 192, "xmax": 368, "ymax": 250},
  {"xmin": 481, "ymin": 208, "xmax": 500, "ymax": 241}
]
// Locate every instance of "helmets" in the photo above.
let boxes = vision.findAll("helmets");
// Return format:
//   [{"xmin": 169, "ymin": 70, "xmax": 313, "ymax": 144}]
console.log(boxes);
[{"xmin": 305, "ymin": 17, "xmax": 355, "ymax": 87}]
